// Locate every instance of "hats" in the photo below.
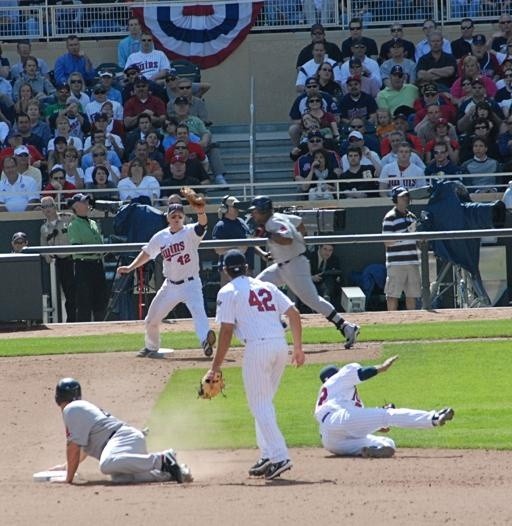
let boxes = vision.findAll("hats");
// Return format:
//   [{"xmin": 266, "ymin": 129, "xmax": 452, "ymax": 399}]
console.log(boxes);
[
  {"xmin": 435, "ymin": 118, "xmax": 448, "ymax": 125},
  {"xmin": 320, "ymin": 365, "xmax": 338, "ymax": 384},
  {"xmin": 471, "ymin": 78, "xmax": 483, "ymax": 86},
  {"xmin": 71, "ymin": 194, "xmax": 90, "ymax": 205},
  {"xmin": 12, "ymin": 232, "xmax": 28, "ymax": 243},
  {"xmin": 391, "ymin": 65, "xmax": 403, "ymax": 75},
  {"xmin": 248, "ymin": 196, "xmax": 273, "ymax": 212},
  {"xmin": 392, "ymin": 186, "xmax": 408, "ymax": 197},
  {"xmin": 224, "ymin": 250, "xmax": 247, "ymax": 267},
  {"xmin": 472, "ymin": 34, "xmax": 486, "ymax": 45},
  {"xmin": 424, "ymin": 84, "xmax": 439, "ymax": 95},
  {"xmin": 168, "ymin": 204, "xmax": 184, "ymax": 215}
]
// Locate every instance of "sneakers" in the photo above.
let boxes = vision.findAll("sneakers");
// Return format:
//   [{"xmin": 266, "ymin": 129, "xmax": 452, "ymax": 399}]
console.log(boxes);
[
  {"xmin": 137, "ymin": 348, "xmax": 152, "ymax": 358},
  {"xmin": 364, "ymin": 445, "xmax": 395, "ymax": 458},
  {"xmin": 250, "ymin": 459, "xmax": 292, "ymax": 479},
  {"xmin": 433, "ymin": 408, "xmax": 454, "ymax": 427},
  {"xmin": 343, "ymin": 324, "xmax": 361, "ymax": 348},
  {"xmin": 160, "ymin": 449, "xmax": 192, "ymax": 482},
  {"xmin": 203, "ymin": 329, "xmax": 216, "ymax": 356}
]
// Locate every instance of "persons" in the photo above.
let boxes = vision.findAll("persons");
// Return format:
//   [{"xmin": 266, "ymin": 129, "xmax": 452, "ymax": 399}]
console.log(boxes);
[
  {"xmin": 246, "ymin": 194, "xmax": 361, "ymax": 349},
  {"xmin": 314, "ymin": 354, "xmax": 454, "ymax": 457},
  {"xmin": 114, "ymin": 196, "xmax": 216, "ymax": 358},
  {"xmin": 266, "ymin": 3, "xmax": 512, "ymax": 308},
  {"xmin": 1, "ymin": 2, "xmax": 255, "ymax": 309},
  {"xmin": 210, "ymin": 249, "xmax": 305, "ymax": 478},
  {"xmin": 55, "ymin": 378, "xmax": 194, "ymax": 485}
]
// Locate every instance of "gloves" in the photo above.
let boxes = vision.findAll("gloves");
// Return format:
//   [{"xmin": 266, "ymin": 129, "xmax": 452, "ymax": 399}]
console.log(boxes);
[{"xmin": 256, "ymin": 227, "xmax": 265, "ymax": 238}]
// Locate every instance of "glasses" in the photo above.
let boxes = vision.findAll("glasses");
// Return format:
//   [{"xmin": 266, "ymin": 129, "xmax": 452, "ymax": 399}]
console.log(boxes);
[
  {"xmin": 306, "ymin": 27, "xmax": 367, "ymax": 143},
  {"xmin": 9, "ymin": 64, "xmax": 190, "ymax": 180}
]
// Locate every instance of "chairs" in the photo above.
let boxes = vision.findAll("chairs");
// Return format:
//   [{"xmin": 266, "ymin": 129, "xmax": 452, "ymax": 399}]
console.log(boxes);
[
  {"xmin": 47, "ymin": 70, "xmax": 56, "ymax": 86},
  {"xmin": 169, "ymin": 59, "xmax": 201, "ymax": 83},
  {"xmin": 96, "ymin": 62, "xmax": 123, "ymax": 75}
]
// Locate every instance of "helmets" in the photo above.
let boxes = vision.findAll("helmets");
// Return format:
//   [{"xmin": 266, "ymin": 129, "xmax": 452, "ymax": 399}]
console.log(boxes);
[{"xmin": 55, "ymin": 378, "xmax": 83, "ymax": 403}]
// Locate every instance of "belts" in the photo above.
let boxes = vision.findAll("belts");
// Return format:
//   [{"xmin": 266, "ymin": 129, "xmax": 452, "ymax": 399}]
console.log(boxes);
[{"xmin": 170, "ymin": 276, "xmax": 194, "ymax": 285}]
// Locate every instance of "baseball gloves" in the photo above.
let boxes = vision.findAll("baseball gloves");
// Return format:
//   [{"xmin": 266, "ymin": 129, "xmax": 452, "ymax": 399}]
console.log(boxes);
[
  {"xmin": 181, "ymin": 186, "xmax": 204, "ymax": 209},
  {"xmin": 197, "ymin": 371, "xmax": 224, "ymax": 399}
]
